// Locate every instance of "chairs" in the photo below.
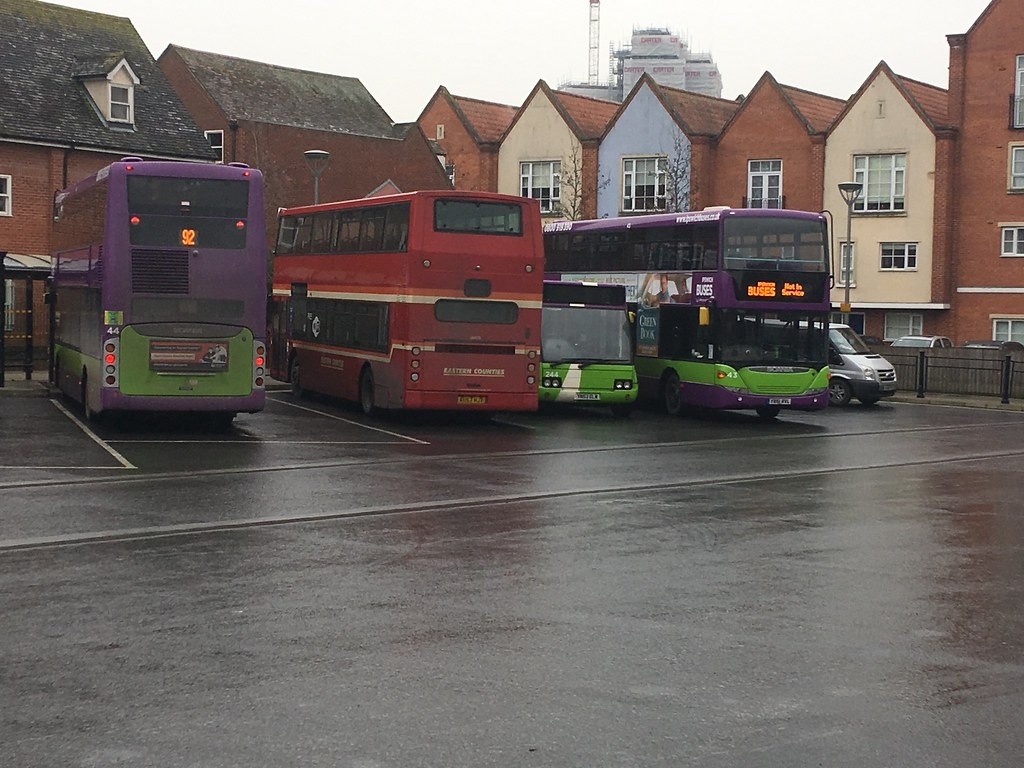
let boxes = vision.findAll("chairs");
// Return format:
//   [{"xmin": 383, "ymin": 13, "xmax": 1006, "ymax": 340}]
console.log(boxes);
[
  {"xmin": 304, "ymin": 224, "xmax": 496, "ymax": 251},
  {"xmin": 551, "ymin": 241, "xmax": 804, "ymax": 271}
]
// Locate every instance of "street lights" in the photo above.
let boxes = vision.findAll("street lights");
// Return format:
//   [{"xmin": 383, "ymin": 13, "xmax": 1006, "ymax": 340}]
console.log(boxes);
[
  {"xmin": 834, "ymin": 182, "xmax": 862, "ymax": 319},
  {"xmin": 303, "ymin": 148, "xmax": 329, "ymax": 204}
]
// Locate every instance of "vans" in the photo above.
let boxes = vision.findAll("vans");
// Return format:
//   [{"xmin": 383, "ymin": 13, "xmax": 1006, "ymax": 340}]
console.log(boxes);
[{"xmin": 742, "ymin": 316, "xmax": 898, "ymax": 407}]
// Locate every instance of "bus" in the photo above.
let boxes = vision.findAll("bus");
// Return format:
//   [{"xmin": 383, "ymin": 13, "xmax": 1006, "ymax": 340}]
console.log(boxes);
[
  {"xmin": 541, "ymin": 205, "xmax": 833, "ymax": 422},
  {"xmin": 51, "ymin": 156, "xmax": 270, "ymax": 421},
  {"xmin": 534, "ymin": 280, "xmax": 640, "ymax": 418},
  {"xmin": 272, "ymin": 189, "xmax": 546, "ymax": 422}
]
